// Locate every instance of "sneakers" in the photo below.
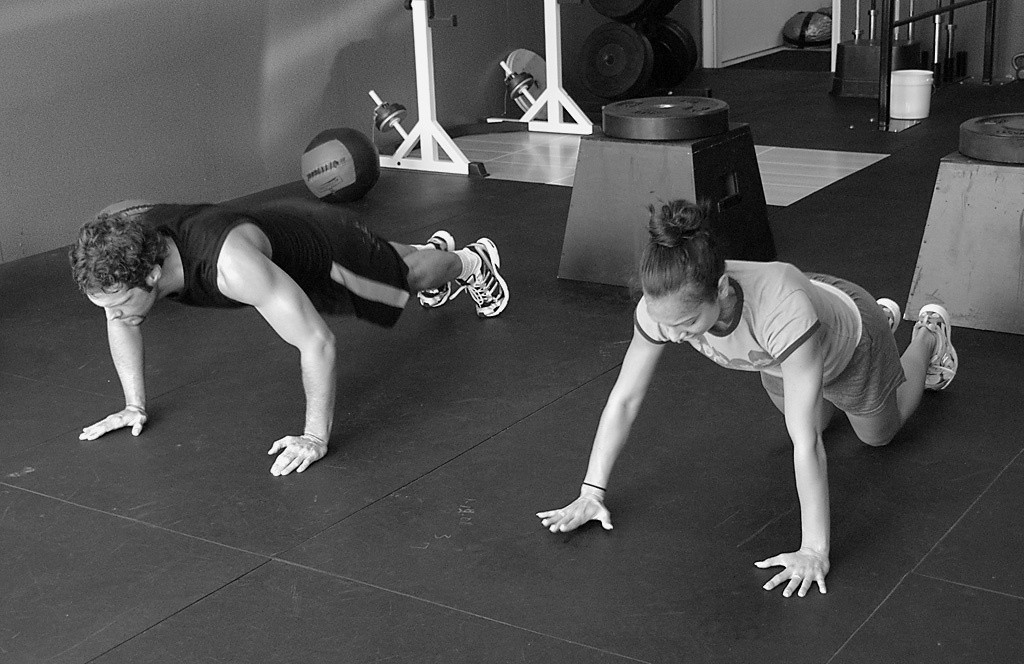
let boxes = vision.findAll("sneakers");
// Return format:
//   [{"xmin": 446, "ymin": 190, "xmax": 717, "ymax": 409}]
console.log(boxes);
[
  {"xmin": 912, "ymin": 304, "xmax": 959, "ymax": 389},
  {"xmin": 874, "ymin": 297, "xmax": 901, "ymax": 334},
  {"xmin": 417, "ymin": 231, "xmax": 457, "ymax": 309},
  {"xmin": 449, "ymin": 237, "xmax": 509, "ymax": 317}
]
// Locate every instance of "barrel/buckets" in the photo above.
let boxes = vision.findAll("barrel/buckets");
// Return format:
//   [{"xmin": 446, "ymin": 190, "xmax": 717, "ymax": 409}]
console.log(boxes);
[{"xmin": 890, "ymin": 70, "xmax": 936, "ymax": 120}]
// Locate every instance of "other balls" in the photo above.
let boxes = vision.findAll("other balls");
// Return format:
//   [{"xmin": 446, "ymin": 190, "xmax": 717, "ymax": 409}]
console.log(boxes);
[{"xmin": 301, "ymin": 128, "xmax": 379, "ymax": 203}]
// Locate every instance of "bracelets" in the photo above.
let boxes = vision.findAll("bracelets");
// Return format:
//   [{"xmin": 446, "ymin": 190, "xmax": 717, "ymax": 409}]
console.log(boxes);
[{"xmin": 582, "ymin": 483, "xmax": 607, "ymax": 492}]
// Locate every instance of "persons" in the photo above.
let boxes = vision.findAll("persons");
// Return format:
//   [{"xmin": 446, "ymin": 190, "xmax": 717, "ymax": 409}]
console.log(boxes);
[
  {"xmin": 69, "ymin": 198, "xmax": 509, "ymax": 476},
  {"xmin": 535, "ymin": 199, "xmax": 958, "ymax": 597}
]
